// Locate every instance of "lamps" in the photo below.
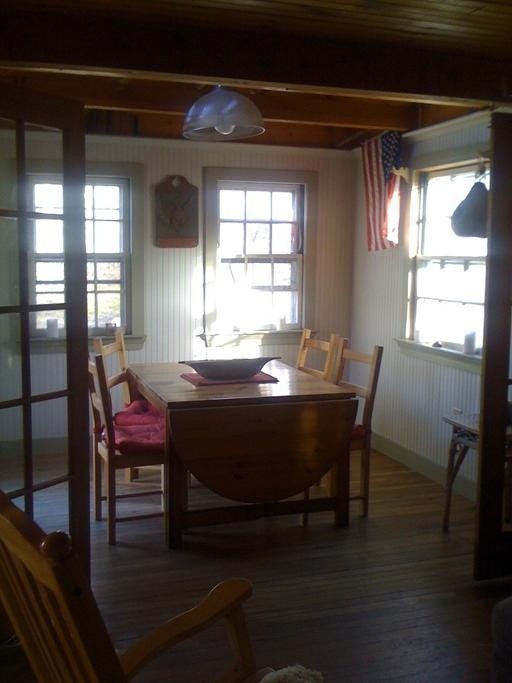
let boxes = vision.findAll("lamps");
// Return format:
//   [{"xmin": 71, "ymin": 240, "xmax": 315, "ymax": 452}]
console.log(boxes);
[{"xmin": 183, "ymin": 85, "xmax": 269, "ymax": 141}]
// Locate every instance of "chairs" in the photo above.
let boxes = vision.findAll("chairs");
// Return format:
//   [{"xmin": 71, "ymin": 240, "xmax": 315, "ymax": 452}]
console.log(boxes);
[
  {"xmin": 84, "ymin": 353, "xmax": 183, "ymax": 541},
  {"xmin": 93, "ymin": 328, "xmax": 135, "ymax": 415},
  {"xmin": 1, "ymin": 489, "xmax": 276, "ymax": 683},
  {"xmin": 300, "ymin": 339, "xmax": 383, "ymax": 529},
  {"xmin": 294, "ymin": 328, "xmax": 337, "ymax": 385}
]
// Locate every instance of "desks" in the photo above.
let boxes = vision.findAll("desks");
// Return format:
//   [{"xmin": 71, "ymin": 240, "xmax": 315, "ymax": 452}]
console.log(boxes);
[{"xmin": 442, "ymin": 413, "xmax": 478, "ymax": 533}]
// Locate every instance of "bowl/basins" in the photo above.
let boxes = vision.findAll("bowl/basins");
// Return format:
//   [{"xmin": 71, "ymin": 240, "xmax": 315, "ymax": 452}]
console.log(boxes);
[{"xmin": 177, "ymin": 357, "xmax": 283, "ymax": 378}]
